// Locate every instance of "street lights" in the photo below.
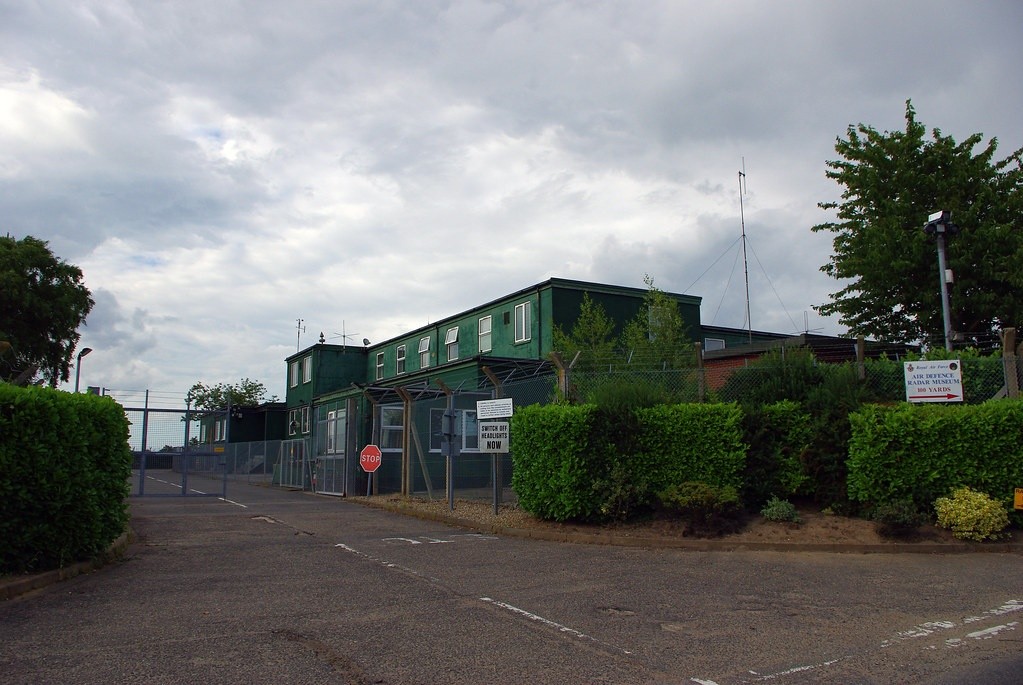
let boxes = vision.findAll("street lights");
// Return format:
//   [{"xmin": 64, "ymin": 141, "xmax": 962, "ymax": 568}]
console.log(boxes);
[
  {"xmin": 75, "ymin": 347, "xmax": 92, "ymax": 395},
  {"xmin": 102, "ymin": 388, "xmax": 111, "ymax": 396},
  {"xmin": 923, "ymin": 209, "xmax": 955, "ymax": 354}
]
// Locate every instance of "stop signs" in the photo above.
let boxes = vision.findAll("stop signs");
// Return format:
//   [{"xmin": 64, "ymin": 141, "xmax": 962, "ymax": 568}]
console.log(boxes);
[{"xmin": 360, "ymin": 445, "xmax": 382, "ymax": 473}]
